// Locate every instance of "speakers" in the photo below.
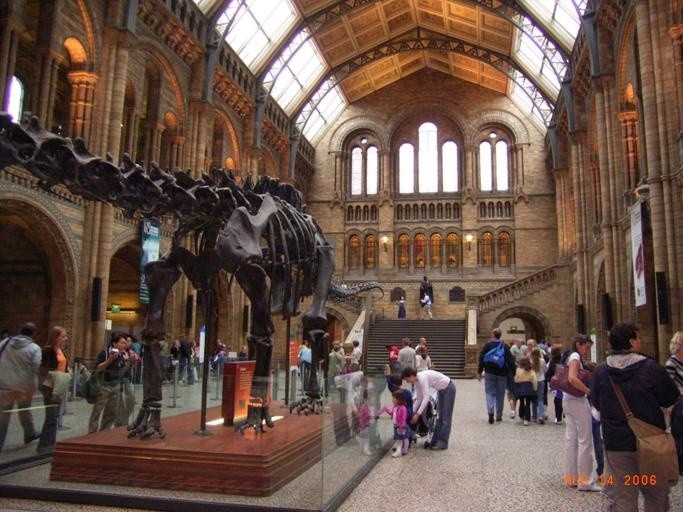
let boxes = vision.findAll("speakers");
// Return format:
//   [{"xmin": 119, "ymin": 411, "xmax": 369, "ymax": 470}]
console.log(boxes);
[
  {"xmin": 656, "ymin": 271, "xmax": 668, "ymax": 325},
  {"xmin": 90, "ymin": 277, "xmax": 102, "ymax": 321},
  {"xmin": 600, "ymin": 293, "xmax": 609, "ymax": 330},
  {"xmin": 577, "ymin": 304, "xmax": 583, "ymax": 333},
  {"xmin": 185, "ymin": 295, "xmax": 193, "ymax": 329},
  {"xmin": 243, "ymin": 305, "xmax": 248, "ymax": 332}
]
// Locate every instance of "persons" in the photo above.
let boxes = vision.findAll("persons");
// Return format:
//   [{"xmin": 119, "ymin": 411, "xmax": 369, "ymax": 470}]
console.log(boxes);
[
  {"xmin": 477, "ymin": 327, "xmax": 566, "ymax": 426},
  {"xmin": 587, "ymin": 326, "xmax": 683, "ymax": 512},
  {"xmin": 327, "ymin": 341, "xmax": 361, "ymax": 399},
  {"xmin": 386, "ymin": 337, "xmax": 457, "ymax": 458},
  {"xmin": 298, "ymin": 340, "xmax": 315, "ymax": 391},
  {"xmin": 168, "ymin": 339, "xmax": 227, "ymax": 384},
  {"xmin": 0, "ymin": 322, "xmax": 69, "ymax": 456},
  {"xmin": 419, "ymin": 276, "xmax": 433, "ymax": 304},
  {"xmin": 71, "ymin": 330, "xmax": 140, "ymax": 433},
  {"xmin": 556, "ymin": 334, "xmax": 609, "ymax": 492}
]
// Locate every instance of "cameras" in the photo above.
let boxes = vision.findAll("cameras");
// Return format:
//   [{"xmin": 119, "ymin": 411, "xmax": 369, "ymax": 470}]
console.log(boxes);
[{"xmin": 111, "ymin": 349, "xmax": 119, "ymax": 355}]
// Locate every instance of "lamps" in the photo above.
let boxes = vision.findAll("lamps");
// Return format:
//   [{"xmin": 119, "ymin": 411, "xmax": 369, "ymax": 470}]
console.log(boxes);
[
  {"xmin": 464, "ymin": 231, "xmax": 473, "ymax": 252},
  {"xmin": 381, "ymin": 232, "xmax": 389, "ymax": 253}
]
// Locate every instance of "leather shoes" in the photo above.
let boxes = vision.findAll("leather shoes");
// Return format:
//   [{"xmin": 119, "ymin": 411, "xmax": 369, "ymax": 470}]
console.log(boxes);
[
  {"xmin": 431, "ymin": 443, "xmax": 446, "ymax": 451},
  {"xmin": 24, "ymin": 430, "xmax": 43, "ymax": 444}
]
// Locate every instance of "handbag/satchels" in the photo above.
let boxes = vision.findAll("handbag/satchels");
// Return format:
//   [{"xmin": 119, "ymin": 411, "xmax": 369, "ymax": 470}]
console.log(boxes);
[
  {"xmin": 603, "ymin": 353, "xmax": 680, "ymax": 490},
  {"xmin": 481, "ymin": 340, "xmax": 507, "ymax": 370},
  {"xmin": 550, "ymin": 352, "xmax": 592, "ymax": 399},
  {"xmin": 80, "ymin": 349, "xmax": 108, "ymax": 404}
]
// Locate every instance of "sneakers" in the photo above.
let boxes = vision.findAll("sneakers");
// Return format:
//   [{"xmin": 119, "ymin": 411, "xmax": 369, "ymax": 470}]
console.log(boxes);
[
  {"xmin": 488, "ymin": 412, "xmax": 565, "ymax": 425},
  {"xmin": 391, "ymin": 451, "xmax": 402, "ymax": 458},
  {"xmin": 576, "ymin": 480, "xmax": 603, "ymax": 493}
]
